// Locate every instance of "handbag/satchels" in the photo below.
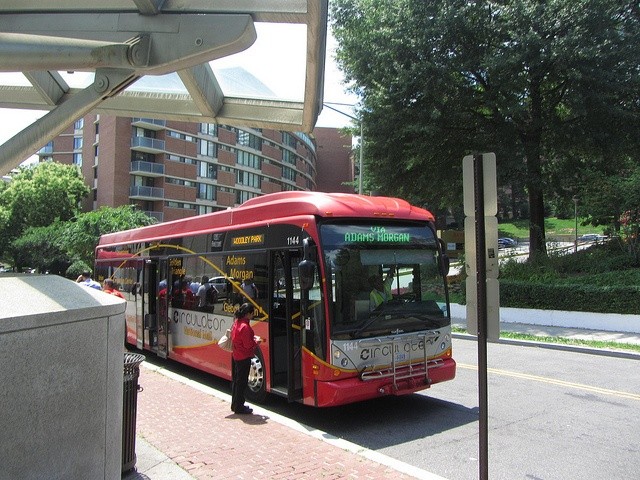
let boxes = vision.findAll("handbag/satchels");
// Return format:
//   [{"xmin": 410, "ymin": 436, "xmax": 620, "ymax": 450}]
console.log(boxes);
[{"xmin": 217, "ymin": 322, "xmax": 233, "ymax": 353}]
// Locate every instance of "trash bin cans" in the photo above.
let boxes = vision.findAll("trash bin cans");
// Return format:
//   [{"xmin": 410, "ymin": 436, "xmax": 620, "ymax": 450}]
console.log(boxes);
[{"xmin": 122, "ymin": 352, "xmax": 146, "ymax": 476}]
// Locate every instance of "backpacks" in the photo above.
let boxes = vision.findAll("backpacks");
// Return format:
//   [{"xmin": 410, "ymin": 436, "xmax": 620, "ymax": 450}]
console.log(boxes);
[{"xmin": 207, "ymin": 284, "xmax": 218, "ymax": 305}]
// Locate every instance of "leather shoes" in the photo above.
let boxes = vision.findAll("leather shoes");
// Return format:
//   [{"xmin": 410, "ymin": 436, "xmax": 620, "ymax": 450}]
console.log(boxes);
[
  {"xmin": 235, "ymin": 409, "xmax": 253, "ymax": 415},
  {"xmin": 231, "ymin": 405, "xmax": 249, "ymax": 412}
]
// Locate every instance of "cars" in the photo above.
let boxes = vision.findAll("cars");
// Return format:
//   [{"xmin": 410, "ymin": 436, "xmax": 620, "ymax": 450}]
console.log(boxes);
[
  {"xmin": 505, "ymin": 238, "xmax": 513, "ymax": 242},
  {"xmin": 504, "ymin": 240, "xmax": 511, "ymax": 247}
]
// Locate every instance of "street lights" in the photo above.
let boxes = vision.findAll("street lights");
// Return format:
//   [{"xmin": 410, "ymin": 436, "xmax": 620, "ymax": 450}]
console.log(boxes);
[{"xmin": 572, "ymin": 195, "xmax": 580, "ymax": 253}]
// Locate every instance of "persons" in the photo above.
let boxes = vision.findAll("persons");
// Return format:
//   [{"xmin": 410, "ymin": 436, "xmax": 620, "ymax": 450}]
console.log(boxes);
[
  {"xmin": 157, "ymin": 273, "xmax": 167, "ymax": 307},
  {"xmin": 196, "ymin": 275, "xmax": 219, "ymax": 313},
  {"xmin": 190, "ymin": 277, "xmax": 202, "ymax": 305},
  {"xmin": 76, "ymin": 269, "xmax": 103, "ymax": 290},
  {"xmin": 102, "ymin": 278, "xmax": 124, "ymax": 299},
  {"xmin": 369, "ymin": 264, "xmax": 395, "ymax": 313},
  {"xmin": 230, "ymin": 302, "xmax": 262, "ymax": 414},
  {"xmin": 240, "ymin": 278, "xmax": 259, "ymax": 303},
  {"xmin": 223, "ymin": 272, "xmax": 243, "ymax": 305},
  {"xmin": 173, "ymin": 279, "xmax": 195, "ymax": 310}
]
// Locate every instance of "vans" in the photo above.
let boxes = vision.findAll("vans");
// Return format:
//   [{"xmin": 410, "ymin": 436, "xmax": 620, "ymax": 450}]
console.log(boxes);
[{"xmin": 582, "ymin": 234, "xmax": 598, "ymax": 240}]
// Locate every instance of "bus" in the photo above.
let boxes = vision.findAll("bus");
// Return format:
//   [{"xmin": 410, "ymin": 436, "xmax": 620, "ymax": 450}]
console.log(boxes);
[{"xmin": 95, "ymin": 191, "xmax": 455, "ymax": 407}]
